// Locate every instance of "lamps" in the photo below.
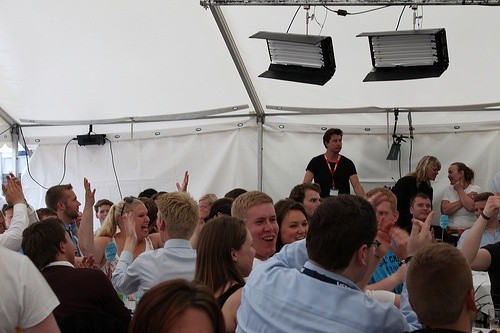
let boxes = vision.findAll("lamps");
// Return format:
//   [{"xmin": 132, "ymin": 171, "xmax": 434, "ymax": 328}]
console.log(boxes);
[
  {"xmin": 356, "ymin": 27, "xmax": 450, "ymax": 82},
  {"xmin": 249, "ymin": 31, "xmax": 336, "ymax": 86}
]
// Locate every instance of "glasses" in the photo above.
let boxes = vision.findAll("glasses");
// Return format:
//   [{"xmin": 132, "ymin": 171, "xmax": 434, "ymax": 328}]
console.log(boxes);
[
  {"xmin": 120, "ymin": 195, "xmax": 138, "ymax": 216},
  {"xmin": 370, "ymin": 240, "xmax": 382, "ymax": 247}
]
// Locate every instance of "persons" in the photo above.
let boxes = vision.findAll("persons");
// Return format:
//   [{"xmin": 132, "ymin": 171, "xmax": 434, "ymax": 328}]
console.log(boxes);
[{"xmin": 0, "ymin": 127, "xmax": 500, "ymax": 333}]
